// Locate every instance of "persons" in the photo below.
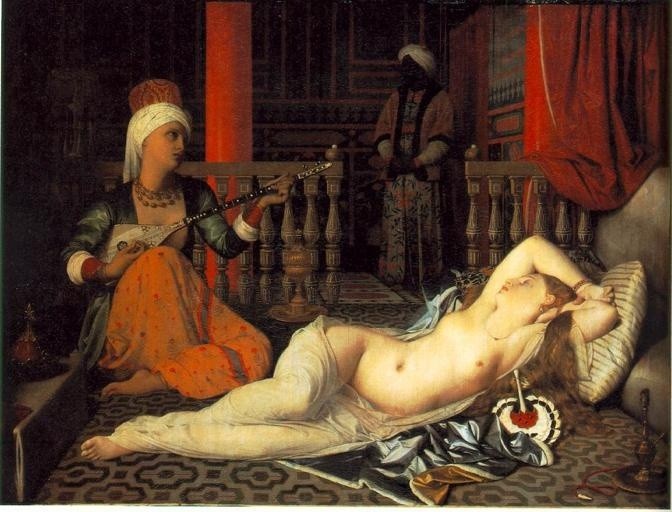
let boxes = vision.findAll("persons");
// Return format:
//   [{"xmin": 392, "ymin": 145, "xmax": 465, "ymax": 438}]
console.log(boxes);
[
  {"xmin": 370, "ymin": 42, "xmax": 455, "ymax": 288},
  {"xmin": 59, "ymin": 77, "xmax": 295, "ymax": 399},
  {"xmin": 79, "ymin": 232, "xmax": 619, "ymax": 467},
  {"xmin": 97, "ymin": 263, "xmax": 108, "ymax": 282}
]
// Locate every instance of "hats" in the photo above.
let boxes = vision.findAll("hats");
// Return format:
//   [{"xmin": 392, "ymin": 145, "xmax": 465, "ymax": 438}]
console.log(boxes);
[
  {"xmin": 398, "ymin": 44, "xmax": 435, "ymax": 71},
  {"xmin": 127, "ymin": 78, "xmax": 193, "ymax": 151}
]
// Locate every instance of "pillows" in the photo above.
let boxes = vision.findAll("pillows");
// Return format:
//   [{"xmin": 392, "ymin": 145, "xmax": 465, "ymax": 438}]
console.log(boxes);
[{"xmin": 538, "ymin": 257, "xmax": 648, "ymax": 405}]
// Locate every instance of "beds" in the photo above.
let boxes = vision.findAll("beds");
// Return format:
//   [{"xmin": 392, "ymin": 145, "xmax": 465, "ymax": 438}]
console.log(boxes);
[{"xmin": 13, "ymin": 165, "xmax": 671, "ymax": 505}]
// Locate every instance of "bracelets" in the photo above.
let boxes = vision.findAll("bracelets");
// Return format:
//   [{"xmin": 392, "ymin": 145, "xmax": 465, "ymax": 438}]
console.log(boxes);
[{"xmin": 571, "ymin": 278, "xmax": 591, "ymax": 293}]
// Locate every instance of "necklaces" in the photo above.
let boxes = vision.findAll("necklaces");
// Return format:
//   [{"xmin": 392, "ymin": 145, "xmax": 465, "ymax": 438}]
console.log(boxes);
[
  {"xmin": 134, "ymin": 179, "xmax": 180, "ymax": 208},
  {"xmin": 485, "ymin": 316, "xmax": 512, "ymax": 343}
]
